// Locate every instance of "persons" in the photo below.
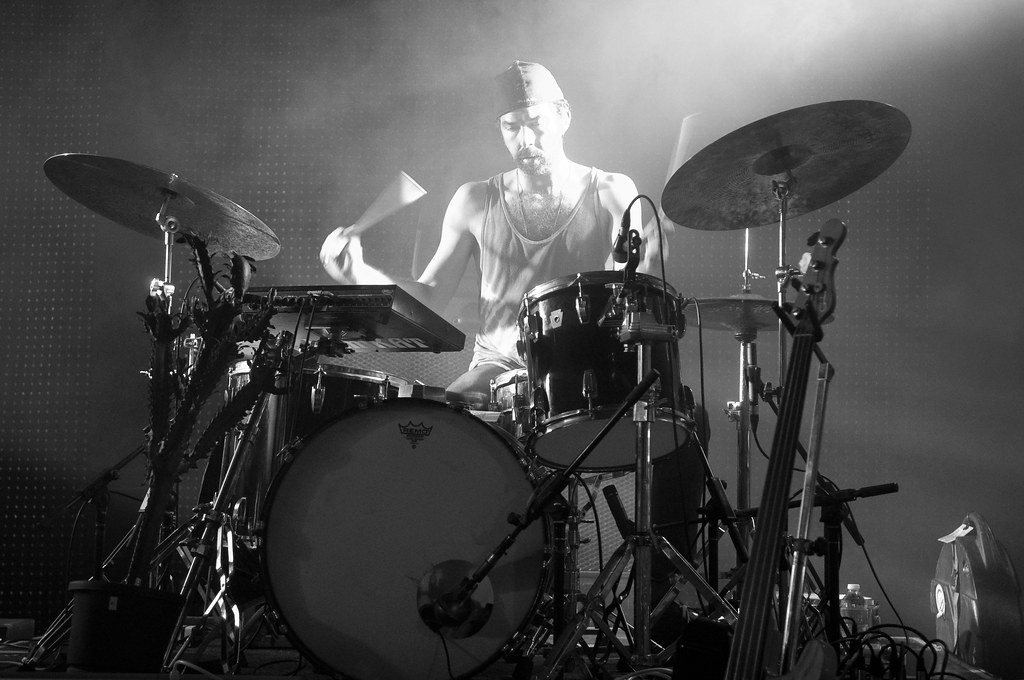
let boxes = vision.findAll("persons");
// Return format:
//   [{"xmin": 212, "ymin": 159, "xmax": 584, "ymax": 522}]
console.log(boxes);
[{"xmin": 320, "ymin": 60, "xmax": 669, "ymax": 423}]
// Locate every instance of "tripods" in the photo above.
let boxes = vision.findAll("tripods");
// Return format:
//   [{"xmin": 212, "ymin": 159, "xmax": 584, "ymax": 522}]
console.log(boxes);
[
  {"xmin": 512, "ymin": 252, "xmax": 744, "ymax": 680},
  {"xmin": 20, "ymin": 230, "xmax": 279, "ymax": 676}
]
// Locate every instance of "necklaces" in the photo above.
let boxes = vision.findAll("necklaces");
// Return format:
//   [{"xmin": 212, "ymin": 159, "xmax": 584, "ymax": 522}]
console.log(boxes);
[{"xmin": 516, "ymin": 169, "xmax": 564, "ymax": 239}]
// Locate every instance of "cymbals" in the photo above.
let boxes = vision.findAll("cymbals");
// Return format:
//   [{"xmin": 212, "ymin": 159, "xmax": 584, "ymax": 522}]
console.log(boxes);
[
  {"xmin": 661, "ymin": 97, "xmax": 912, "ymax": 232},
  {"xmin": 679, "ymin": 295, "xmax": 792, "ymax": 332},
  {"xmin": 45, "ymin": 152, "xmax": 282, "ymax": 261}
]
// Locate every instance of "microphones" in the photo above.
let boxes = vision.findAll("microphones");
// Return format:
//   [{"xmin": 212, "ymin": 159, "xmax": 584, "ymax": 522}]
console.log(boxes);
[
  {"xmin": 433, "ymin": 592, "xmax": 474, "ymax": 630},
  {"xmin": 601, "ymin": 483, "xmax": 636, "ymax": 541},
  {"xmin": 612, "ymin": 209, "xmax": 631, "ymax": 264}
]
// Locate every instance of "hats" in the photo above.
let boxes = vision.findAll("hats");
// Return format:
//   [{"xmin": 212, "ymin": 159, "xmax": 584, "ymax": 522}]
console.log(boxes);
[{"xmin": 493, "ymin": 60, "xmax": 563, "ymax": 120}]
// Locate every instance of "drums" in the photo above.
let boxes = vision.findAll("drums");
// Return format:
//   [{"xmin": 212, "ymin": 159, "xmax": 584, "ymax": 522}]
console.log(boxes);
[
  {"xmin": 260, "ymin": 400, "xmax": 583, "ymax": 680},
  {"xmin": 488, "ymin": 367, "xmax": 529, "ymax": 411},
  {"xmin": 271, "ymin": 360, "xmax": 409, "ymax": 465},
  {"xmin": 518, "ymin": 273, "xmax": 694, "ymax": 468}
]
